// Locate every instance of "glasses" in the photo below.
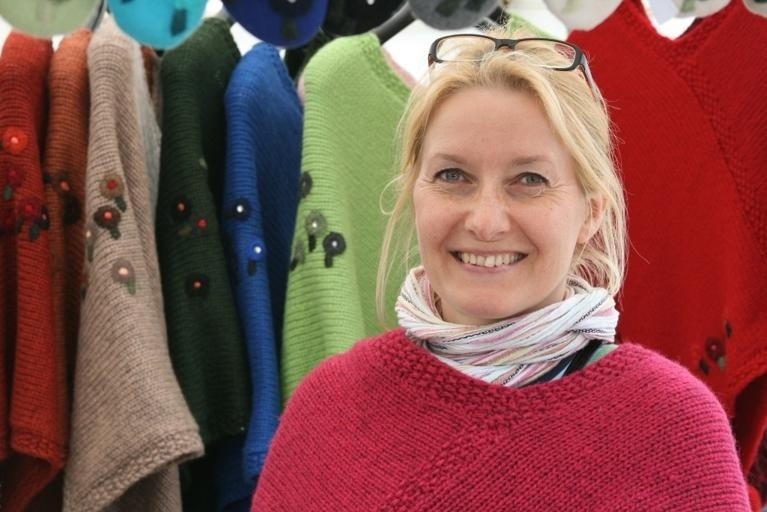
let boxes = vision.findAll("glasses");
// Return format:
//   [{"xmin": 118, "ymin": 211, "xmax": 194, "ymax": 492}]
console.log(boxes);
[{"xmin": 427, "ymin": 34, "xmax": 602, "ymax": 97}]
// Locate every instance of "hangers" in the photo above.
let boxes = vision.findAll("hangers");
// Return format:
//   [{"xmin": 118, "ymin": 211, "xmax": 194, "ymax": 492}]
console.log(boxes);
[
  {"xmin": 83, "ymin": 0, "xmax": 107, "ymax": 31},
  {"xmin": 214, "ymin": 4, "xmax": 235, "ymax": 30},
  {"xmin": 369, "ymin": 0, "xmax": 511, "ymax": 45}
]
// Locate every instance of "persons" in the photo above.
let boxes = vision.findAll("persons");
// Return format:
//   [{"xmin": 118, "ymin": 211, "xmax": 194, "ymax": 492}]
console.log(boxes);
[{"xmin": 250, "ymin": 27, "xmax": 752, "ymax": 512}]
